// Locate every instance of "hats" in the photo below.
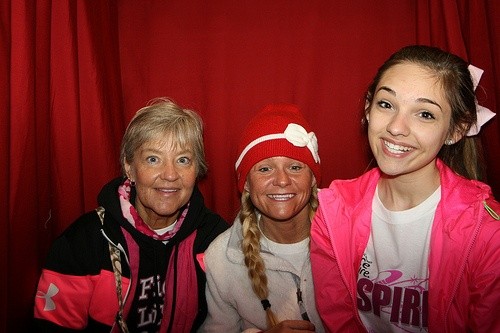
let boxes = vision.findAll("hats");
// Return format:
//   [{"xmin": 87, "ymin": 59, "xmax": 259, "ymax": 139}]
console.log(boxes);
[{"xmin": 235, "ymin": 104, "xmax": 321, "ymax": 194}]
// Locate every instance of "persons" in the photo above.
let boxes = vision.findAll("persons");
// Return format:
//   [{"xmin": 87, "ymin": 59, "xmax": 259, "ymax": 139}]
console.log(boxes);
[
  {"xmin": 33, "ymin": 96, "xmax": 231, "ymax": 333},
  {"xmin": 311, "ymin": 44, "xmax": 500, "ymax": 333},
  {"xmin": 197, "ymin": 102, "xmax": 321, "ymax": 333}
]
